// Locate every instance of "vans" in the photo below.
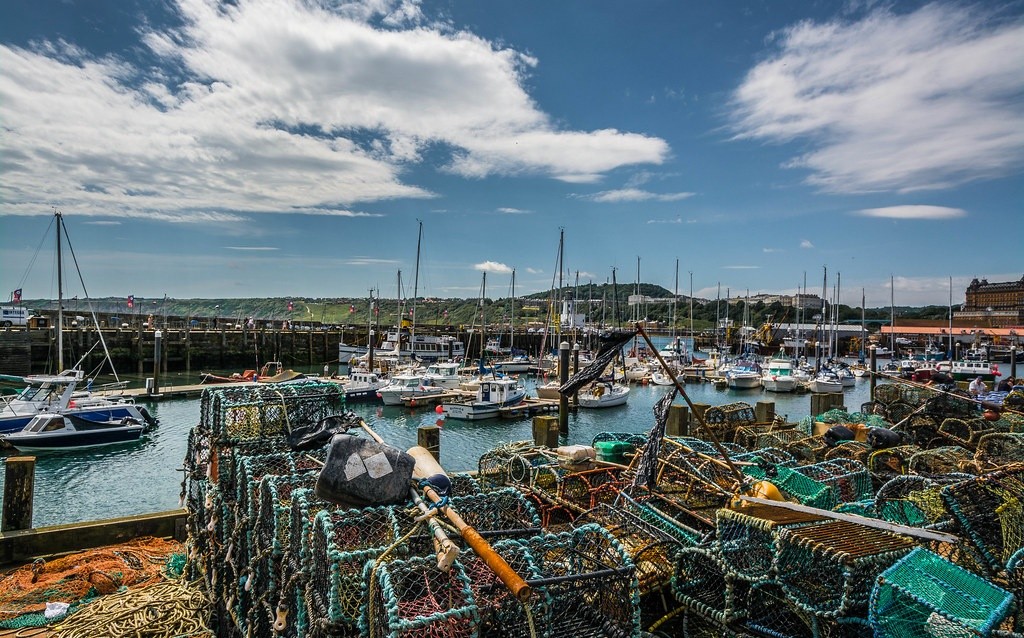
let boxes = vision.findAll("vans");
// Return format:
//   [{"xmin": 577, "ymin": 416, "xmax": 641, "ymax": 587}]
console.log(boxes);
[{"xmin": 0, "ymin": 305, "xmax": 28, "ymax": 328}]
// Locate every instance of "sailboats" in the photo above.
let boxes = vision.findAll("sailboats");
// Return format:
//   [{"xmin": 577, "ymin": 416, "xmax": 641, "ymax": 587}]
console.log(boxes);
[
  {"xmin": 577, "ymin": 264, "xmax": 630, "ymax": 407},
  {"xmin": 0, "ymin": 206, "xmax": 154, "ymax": 443},
  {"xmin": 380, "ymin": 218, "xmax": 445, "ymax": 405},
  {"xmin": 519, "ymin": 225, "xmax": 957, "ymax": 407},
  {"xmin": 459, "ymin": 263, "xmax": 538, "ymax": 378}
]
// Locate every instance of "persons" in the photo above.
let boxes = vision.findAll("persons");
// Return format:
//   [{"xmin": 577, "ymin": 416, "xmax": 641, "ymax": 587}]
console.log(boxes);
[
  {"xmin": 969, "ymin": 376, "xmax": 987, "ymax": 395},
  {"xmin": 212, "ymin": 316, "xmax": 217, "ymax": 330},
  {"xmin": 998, "ymin": 376, "xmax": 1014, "ymax": 392},
  {"xmin": 244, "ymin": 316, "xmax": 253, "ymax": 329},
  {"xmin": 348, "ymin": 359, "xmax": 353, "ymax": 376},
  {"xmin": 324, "ymin": 364, "xmax": 328, "ymax": 379},
  {"xmin": 282, "ymin": 319, "xmax": 292, "ymax": 330},
  {"xmin": 331, "ymin": 370, "xmax": 340, "ymax": 379},
  {"xmin": 148, "ymin": 315, "xmax": 153, "ymax": 329}
]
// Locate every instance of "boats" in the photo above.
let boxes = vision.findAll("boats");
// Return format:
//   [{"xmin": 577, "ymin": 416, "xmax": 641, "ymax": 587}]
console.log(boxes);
[
  {"xmin": 984, "ymin": 343, "xmax": 1024, "ymax": 362},
  {"xmin": 947, "ymin": 357, "xmax": 995, "ymax": 391},
  {"xmin": 423, "ymin": 362, "xmax": 465, "ymax": 390},
  {"xmin": 342, "ymin": 360, "xmax": 391, "ymax": 399},
  {"xmin": 0, "ymin": 403, "xmax": 144, "ymax": 452},
  {"xmin": 441, "ymin": 366, "xmax": 529, "ymax": 421},
  {"xmin": 339, "ymin": 320, "xmax": 464, "ymax": 365},
  {"xmin": 199, "ymin": 360, "xmax": 321, "ymax": 385}
]
